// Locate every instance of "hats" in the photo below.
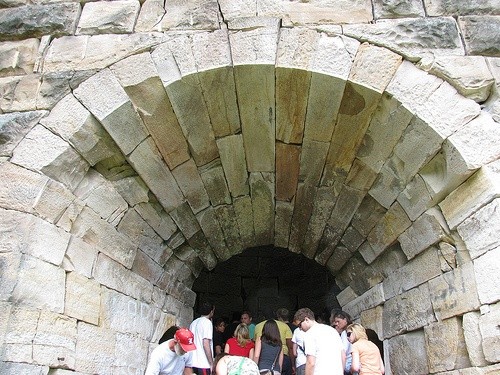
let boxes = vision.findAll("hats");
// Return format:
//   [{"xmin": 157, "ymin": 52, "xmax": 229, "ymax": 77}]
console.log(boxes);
[{"xmin": 175, "ymin": 328, "xmax": 196, "ymax": 352}]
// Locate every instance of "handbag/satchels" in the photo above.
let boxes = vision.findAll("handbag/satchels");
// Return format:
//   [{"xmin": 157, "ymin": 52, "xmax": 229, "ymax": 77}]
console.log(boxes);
[{"xmin": 259, "ymin": 369, "xmax": 272, "ymax": 375}]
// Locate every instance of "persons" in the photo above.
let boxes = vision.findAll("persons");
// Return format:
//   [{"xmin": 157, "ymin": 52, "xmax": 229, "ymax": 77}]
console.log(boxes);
[
  {"xmin": 346, "ymin": 323, "xmax": 384, "ymax": 375},
  {"xmin": 187, "ymin": 301, "xmax": 214, "ymax": 374},
  {"xmin": 255, "ymin": 319, "xmax": 282, "ymax": 375},
  {"xmin": 256, "ymin": 306, "xmax": 295, "ymax": 375},
  {"xmin": 225, "ymin": 324, "xmax": 255, "ymax": 359},
  {"xmin": 240, "ymin": 312, "xmax": 256, "ymax": 340},
  {"xmin": 212, "ymin": 321, "xmax": 227, "ymax": 356},
  {"xmin": 295, "ymin": 308, "xmax": 345, "ymax": 374},
  {"xmin": 291, "ymin": 317, "xmax": 322, "ymax": 375},
  {"xmin": 144, "ymin": 325, "xmax": 195, "ymax": 374},
  {"xmin": 213, "ymin": 353, "xmax": 261, "ymax": 375},
  {"xmin": 334, "ymin": 313, "xmax": 354, "ymax": 371},
  {"xmin": 327, "ymin": 310, "xmax": 341, "ymax": 326}
]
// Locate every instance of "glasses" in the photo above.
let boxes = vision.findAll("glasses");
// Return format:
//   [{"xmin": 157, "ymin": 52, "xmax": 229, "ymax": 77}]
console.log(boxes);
[
  {"xmin": 347, "ymin": 331, "xmax": 353, "ymax": 337},
  {"xmin": 296, "ymin": 321, "xmax": 302, "ymax": 328}
]
